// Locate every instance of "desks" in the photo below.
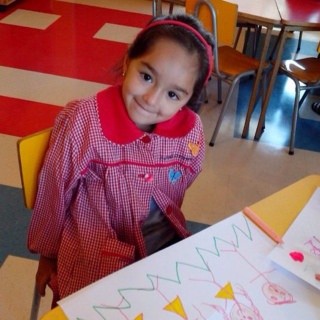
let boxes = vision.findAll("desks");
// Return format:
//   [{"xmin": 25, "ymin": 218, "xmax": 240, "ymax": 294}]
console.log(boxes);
[
  {"xmin": 38, "ymin": 174, "xmax": 320, "ymax": 320},
  {"xmin": 153, "ymin": 0, "xmax": 320, "ymax": 142}
]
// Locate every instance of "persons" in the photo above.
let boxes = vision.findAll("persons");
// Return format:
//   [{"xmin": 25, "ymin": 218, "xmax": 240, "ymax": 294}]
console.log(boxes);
[{"xmin": 27, "ymin": 11, "xmax": 216, "ymax": 303}]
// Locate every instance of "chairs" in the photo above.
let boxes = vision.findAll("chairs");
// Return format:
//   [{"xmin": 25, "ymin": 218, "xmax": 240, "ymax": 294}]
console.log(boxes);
[
  {"xmin": 17, "ymin": 128, "xmax": 61, "ymax": 320},
  {"xmin": 185, "ymin": 0, "xmax": 271, "ymax": 147},
  {"xmin": 269, "ymin": 42, "xmax": 320, "ymax": 155}
]
